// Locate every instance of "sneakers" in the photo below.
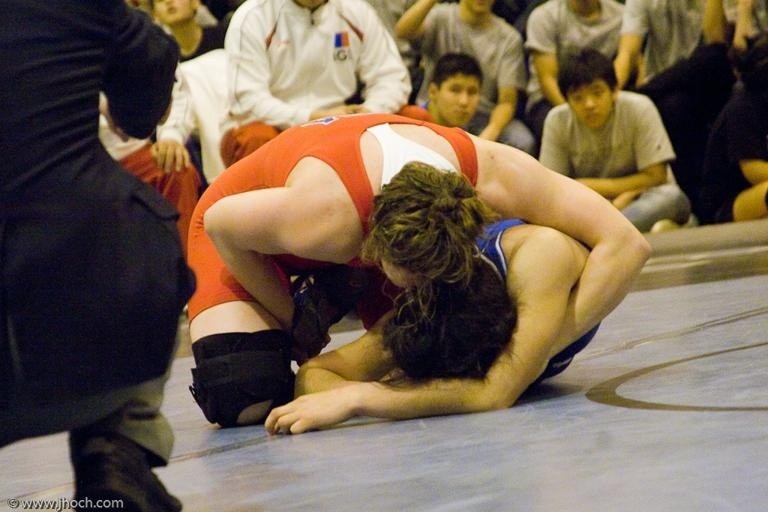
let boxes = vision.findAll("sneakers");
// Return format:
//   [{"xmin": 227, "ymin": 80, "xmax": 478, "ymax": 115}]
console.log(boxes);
[{"xmin": 76, "ymin": 448, "xmax": 181, "ymax": 512}]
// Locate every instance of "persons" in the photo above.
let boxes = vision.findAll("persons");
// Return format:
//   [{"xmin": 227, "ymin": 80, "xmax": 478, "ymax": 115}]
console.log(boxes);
[
  {"xmin": 95, "ymin": 0, "xmax": 768, "ymax": 254},
  {"xmin": 261, "ymin": 216, "xmax": 604, "ymax": 437},
  {"xmin": 0, "ymin": 2, "xmax": 200, "ymax": 512},
  {"xmin": 183, "ymin": 109, "xmax": 654, "ymax": 434}
]
